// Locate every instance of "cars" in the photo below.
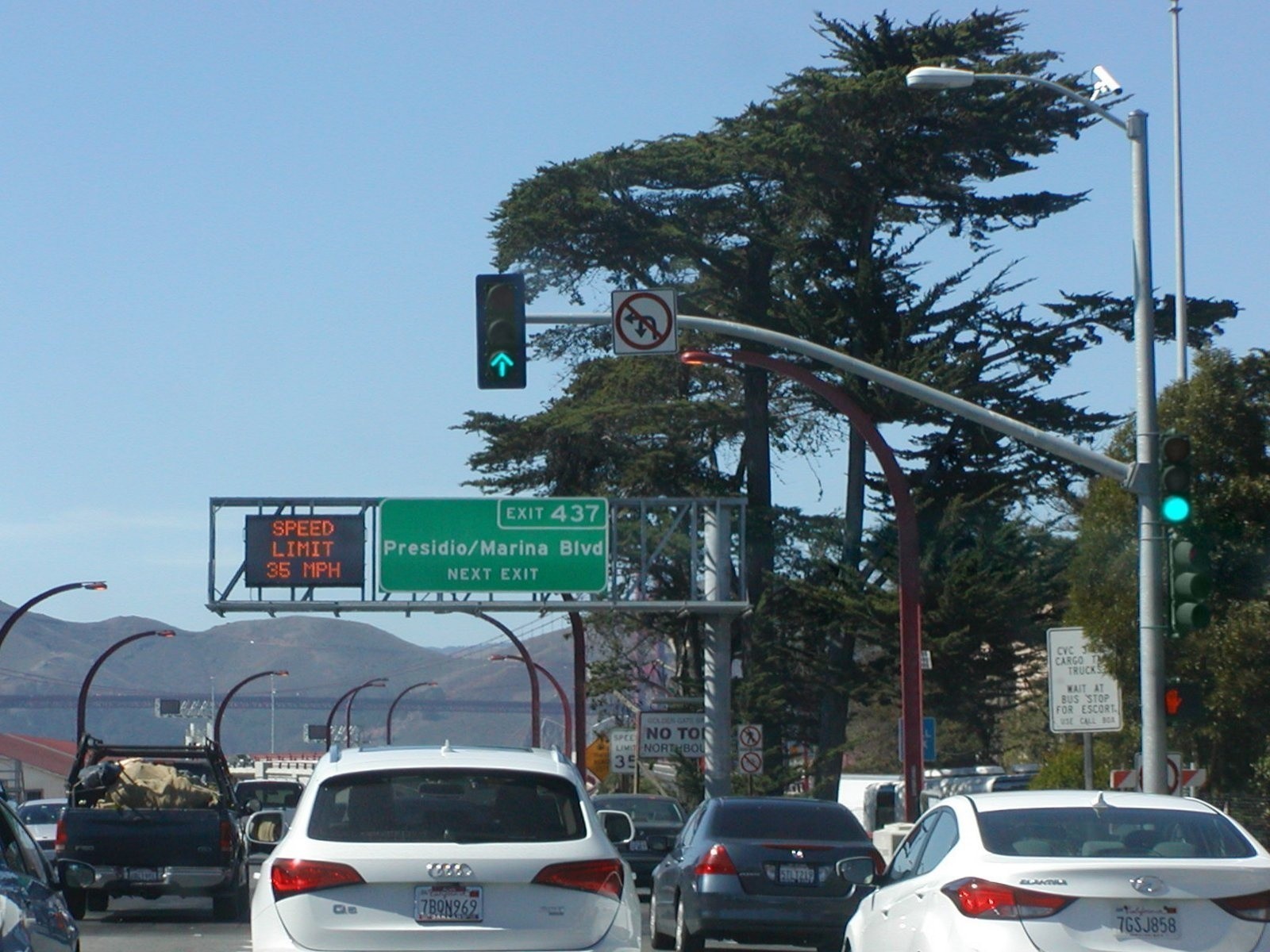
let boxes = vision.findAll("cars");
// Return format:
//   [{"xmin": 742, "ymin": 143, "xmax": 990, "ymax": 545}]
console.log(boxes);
[
  {"xmin": 650, "ymin": 795, "xmax": 888, "ymax": 951},
  {"xmin": 0, "ymin": 796, "xmax": 98, "ymax": 952},
  {"xmin": 836, "ymin": 788, "xmax": 1270, "ymax": 952},
  {"xmin": 587, "ymin": 794, "xmax": 688, "ymax": 888}
]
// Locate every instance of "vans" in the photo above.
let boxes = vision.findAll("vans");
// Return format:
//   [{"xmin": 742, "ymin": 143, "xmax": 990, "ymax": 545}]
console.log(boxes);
[{"xmin": 17, "ymin": 796, "xmax": 87, "ymax": 867}]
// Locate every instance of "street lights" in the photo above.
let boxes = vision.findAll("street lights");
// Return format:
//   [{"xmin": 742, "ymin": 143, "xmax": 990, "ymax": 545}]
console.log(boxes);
[
  {"xmin": 77, "ymin": 627, "xmax": 179, "ymax": 756},
  {"xmin": 325, "ymin": 678, "xmax": 390, "ymax": 754},
  {"xmin": 387, "ymin": 681, "xmax": 437, "ymax": 746},
  {"xmin": 907, "ymin": 62, "xmax": 1167, "ymax": 795},
  {"xmin": 680, "ymin": 351, "xmax": 923, "ymax": 825},
  {"xmin": 488, "ymin": 654, "xmax": 573, "ymax": 759},
  {"xmin": 215, "ymin": 670, "xmax": 289, "ymax": 743}
]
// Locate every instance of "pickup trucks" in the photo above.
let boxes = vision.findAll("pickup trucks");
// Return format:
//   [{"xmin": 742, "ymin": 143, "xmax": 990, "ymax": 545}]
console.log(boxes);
[{"xmin": 54, "ymin": 734, "xmax": 252, "ymax": 924}]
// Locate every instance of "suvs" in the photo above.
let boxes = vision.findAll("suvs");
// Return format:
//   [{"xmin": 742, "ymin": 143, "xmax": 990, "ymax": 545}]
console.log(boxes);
[
  {"xmin": 251, "ymin": 746, "xmax": 643, "ymax": 951},
  {"xmin": 233, "ymin": 778, "xmax": 303, "ymax": 859}
]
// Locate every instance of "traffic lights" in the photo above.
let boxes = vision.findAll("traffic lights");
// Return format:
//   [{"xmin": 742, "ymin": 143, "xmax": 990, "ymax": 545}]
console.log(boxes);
[
  {"xmin": 1165, "ymin": 673, "xmax": 1200, "ymax": 719},
  {"xmin": 1158, "ymin": 431, "xmax": 1190, "ymax": 525},
  {"xmin": 475, "ymin": 274, "xmax": 527, "ymax": 389},
  {"xmin": 1169, "ymin": 536, "xmax": 1211, "ymax": 636}
]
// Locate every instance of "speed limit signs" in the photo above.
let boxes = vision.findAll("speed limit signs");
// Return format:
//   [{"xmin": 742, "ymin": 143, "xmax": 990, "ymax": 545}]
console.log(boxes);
[{"xmin": 609, "ymin": 729, "xmax": 636, "ymax": 774}]
